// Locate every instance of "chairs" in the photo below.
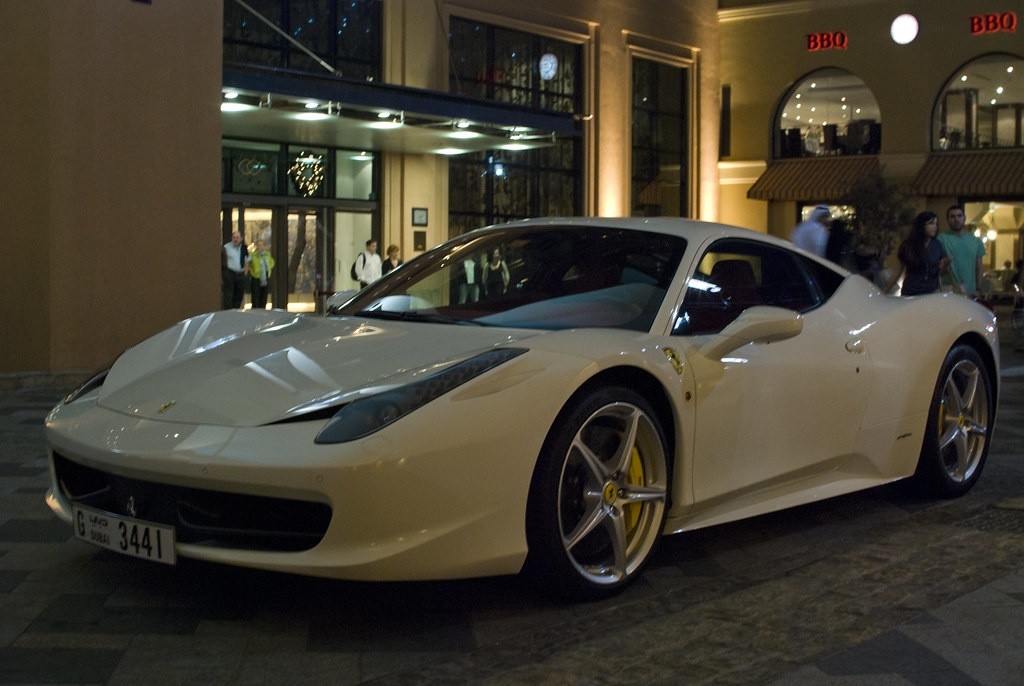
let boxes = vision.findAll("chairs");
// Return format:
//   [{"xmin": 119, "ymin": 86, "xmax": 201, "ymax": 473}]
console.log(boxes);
[
  {"xmin": 781, "ymin": 119, "xmax": 882, "ymax": 158},
  {"xmin": 701, "ymin": 259, "xmax": 755, "ymax": 303}
]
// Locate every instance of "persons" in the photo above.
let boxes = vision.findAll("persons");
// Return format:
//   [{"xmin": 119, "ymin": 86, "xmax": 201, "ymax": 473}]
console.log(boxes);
[
  {"xmin": 482, "ymin": 246, "xmax": 510, "ymax": 297},
  {"xmin": 937, "ymin": 205, "xmax": 986, "ymax": 298},
  {"xmin": 222, "ymin": 231, "xmax": 251, "ymax": 310},
  {"xmin": 354, "ymin": 240, "xmax": 382, "ymax": 291},
  {"xmin": 453, "ymin": 253, "xmax": 481, "ymax": 304},
  {"xmin": 999, "ymin": 260, "xmax": 1024, "ymax": 289},
  {"xmin": 382, "ymin": 245, "xmax": 403, "ymax": 275},
  {"xmin": 247, "ymin": 240, "xmax": 276, "ymax": 309},
  {"xmin": 884, "ymin": 212, "xmax": 948, "ymax": 296},
  {"xmin": 793, "ymin": 206, "xmax": 896, "ymax": 293}
]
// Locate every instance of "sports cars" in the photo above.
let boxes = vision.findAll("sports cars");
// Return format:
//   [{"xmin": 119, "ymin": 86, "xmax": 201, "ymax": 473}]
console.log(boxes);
[{"xmin": 43, "ymin": 215, "xmax": 1002, "ymax": 606}]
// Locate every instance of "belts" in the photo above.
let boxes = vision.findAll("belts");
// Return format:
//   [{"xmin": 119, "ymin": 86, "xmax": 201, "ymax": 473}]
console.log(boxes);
[{"xmin": 228, "ymin": 269, "xmax": 245, "ymax": 275}]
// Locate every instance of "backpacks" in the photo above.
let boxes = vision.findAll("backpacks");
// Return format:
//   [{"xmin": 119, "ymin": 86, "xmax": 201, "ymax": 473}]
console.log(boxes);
[{"xmin": 350, "ymin": 252, "xmax": 366, "ymax": 281}]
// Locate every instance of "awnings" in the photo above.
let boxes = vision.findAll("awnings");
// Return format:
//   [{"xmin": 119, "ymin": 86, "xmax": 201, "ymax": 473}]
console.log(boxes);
[
  {"xmin": 746, "ymin": 158, "xmax": 878, "ymax": 200},
  {"xmin": 909, "ymin": 153, "xmax": 1024, "ymax": 196}
]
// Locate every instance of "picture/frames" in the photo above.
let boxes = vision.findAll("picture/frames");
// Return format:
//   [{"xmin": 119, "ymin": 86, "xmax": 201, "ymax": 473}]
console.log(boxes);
[{"xmin": 412, "ymin": 208, "xmax": 429, "ymax": 227}]
